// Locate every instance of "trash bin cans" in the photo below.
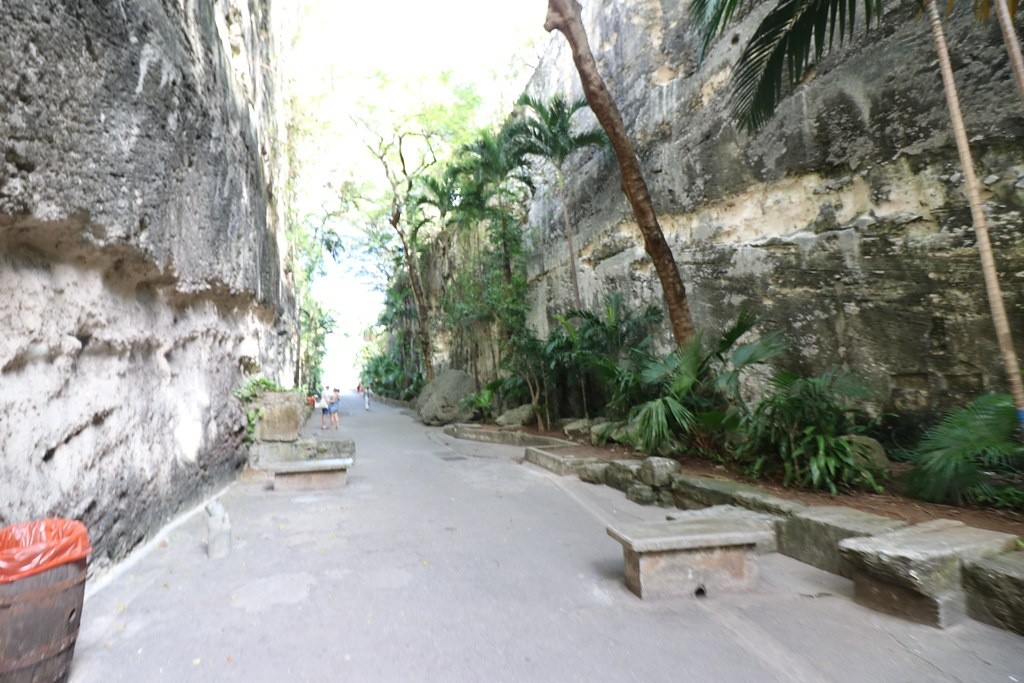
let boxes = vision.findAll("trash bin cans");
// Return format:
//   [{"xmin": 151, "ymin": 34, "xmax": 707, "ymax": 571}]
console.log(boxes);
[{"xmin": 0, "ymin": 519, "xmax": 88, "ymax": 682}]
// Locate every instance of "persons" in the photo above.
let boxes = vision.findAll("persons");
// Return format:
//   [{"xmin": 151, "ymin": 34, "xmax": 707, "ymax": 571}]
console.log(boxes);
[
  {"xmin": 306, "ymin": 393, "xmax": 316, "ymax": 409},
  {"xmin": 321, "ymin": 385, "xmax": 340, "ymax": 431},
  {"xmin": 362, "ymin": 386, "xmax": 374, "ymax": 411}
]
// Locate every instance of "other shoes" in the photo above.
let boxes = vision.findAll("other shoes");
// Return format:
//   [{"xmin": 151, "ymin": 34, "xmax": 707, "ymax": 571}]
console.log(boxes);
[{"xmin": 322, "ymin": 424, "xmax": 330, "ymax": 430}]
